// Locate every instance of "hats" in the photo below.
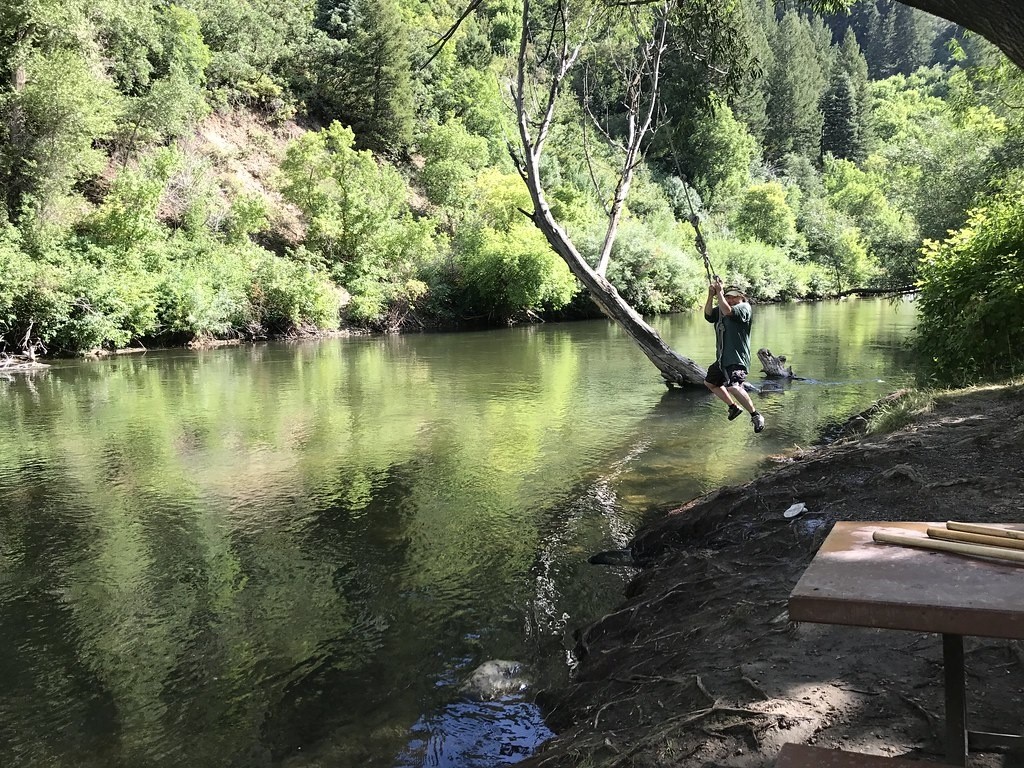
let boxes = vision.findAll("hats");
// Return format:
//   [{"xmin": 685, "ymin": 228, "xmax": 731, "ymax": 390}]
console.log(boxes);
[{"xmin": 724, "ymin": 285, "xmax": 746, "ymax": 297}]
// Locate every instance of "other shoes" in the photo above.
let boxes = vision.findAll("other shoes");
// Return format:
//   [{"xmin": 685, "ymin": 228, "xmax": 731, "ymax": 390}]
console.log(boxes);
[
  {"xmin": 727, "ymin": 404, "xmax": 743, "ymax": 421},
  {"xmin": 751, "ymin": 412, "xmax": 766, "ymax": 433}
]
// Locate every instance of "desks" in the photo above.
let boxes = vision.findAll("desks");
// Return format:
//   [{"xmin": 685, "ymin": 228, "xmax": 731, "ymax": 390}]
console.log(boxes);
[{"xmin": 788, "ymin": 521, "xmax": 1024, "ymax": 768}]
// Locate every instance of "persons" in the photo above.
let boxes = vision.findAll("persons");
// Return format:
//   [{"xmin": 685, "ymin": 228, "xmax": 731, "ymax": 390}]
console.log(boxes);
[{"xmin": 703, "ymin": 277, "xmax": 764, "ymax": 434}]
